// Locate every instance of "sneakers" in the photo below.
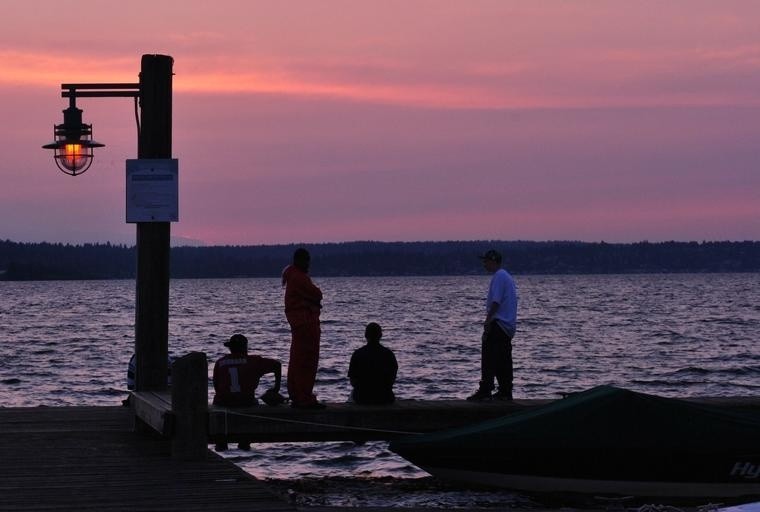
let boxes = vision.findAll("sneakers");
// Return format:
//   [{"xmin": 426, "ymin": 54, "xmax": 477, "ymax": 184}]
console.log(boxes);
[{"xmin": 464, "ymin": 388, "xmax": 515, "ymax": 404}]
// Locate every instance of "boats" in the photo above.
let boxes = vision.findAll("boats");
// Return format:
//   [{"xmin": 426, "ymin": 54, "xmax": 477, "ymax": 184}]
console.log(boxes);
[{"xmin": 387, "ymin": 444, "xmax": 760, "ymax": 509}]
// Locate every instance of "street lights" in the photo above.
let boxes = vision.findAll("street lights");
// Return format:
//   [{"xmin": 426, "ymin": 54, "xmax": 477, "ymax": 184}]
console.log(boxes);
[{"xmin": 41, "ymin": 54, "xmax": 173, "ymax": 390}]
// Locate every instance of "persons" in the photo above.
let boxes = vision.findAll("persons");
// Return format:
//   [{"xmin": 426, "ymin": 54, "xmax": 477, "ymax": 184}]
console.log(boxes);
[
  {"xmin": 210, "ymin": 333, "xmax": 282, "ymax": 451},
  {"xmin": 345, "ymin": 321, "xmax": 399, "ymax": 406},
  {"xmin": 464, "ymin": 248, "xmax": 520, "ymax": 402},
  {"xmin": 119, "ymin": 348, "xmax": 173, "ymax": 407},
  {"xmin": 278, "ymin": 246, "xmax": 327, "ymax": 409}
]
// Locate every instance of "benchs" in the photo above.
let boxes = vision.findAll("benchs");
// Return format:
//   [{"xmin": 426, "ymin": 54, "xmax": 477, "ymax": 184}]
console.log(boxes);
[{"xmin": 126, "ymin": 389, "xmax": 173, "ymax": 438}]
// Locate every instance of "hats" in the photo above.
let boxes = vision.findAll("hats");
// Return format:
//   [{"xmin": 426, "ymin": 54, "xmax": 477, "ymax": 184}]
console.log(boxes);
[
  {"xmin": 477, "ymin": 249, "xmax": 504, "ymax": 265},
  {"xmin": 222, "ymin": 334, "xmax": 250, "ymax": 350}
]
